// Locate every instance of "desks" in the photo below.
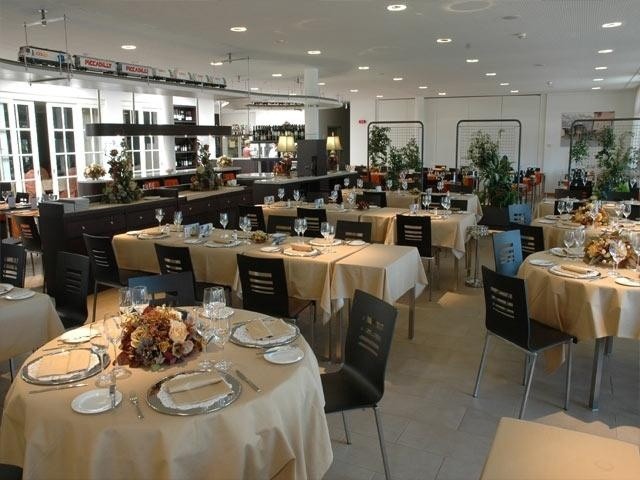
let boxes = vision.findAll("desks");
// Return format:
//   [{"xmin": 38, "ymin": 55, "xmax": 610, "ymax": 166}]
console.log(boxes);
[
  {"xmin": 1, "ymin": 166, "xmax": 250, "ymax": 250},
  {"xmin": 250, "ymin": 197, "xmax": 477, "ymax": 294},
  {"xmin": 514, "ymin": 246, "xmax": 640, "ymax": 412},
  {"xmin": 113, "ymin": 225, "xmax": 437, "ymax": 341},
  {"xmin": 2, "ymin": 301, "xmax": 334, "ymax": 480}
]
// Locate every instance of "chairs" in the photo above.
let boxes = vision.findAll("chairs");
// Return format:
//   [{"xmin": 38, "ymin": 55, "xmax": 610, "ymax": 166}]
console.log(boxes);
[
  {"xmin": 335, "ymin": 219, "xmax": 373, "ymax": 245},
  {"xmin": 9, "ymin": 251, "xmax": 90, "ymax": 383},
  {"xmin": 395, "ymin": 214, "xmax": 436, "ymax": 302},
  {"xmin": 493, "ymin": 228, "xmax": 523, "ymax": 282},
  {"xmin": 236, "ymin": 252, "xmax": 317, "ymax": 360},
  {"xmin": 319, "ymin": 283, "xmax": 399, "ymax": 480},
  {"xmin": 472, "ymin": 264, "xmax": 580, "ymax": 421},
  {"xmin": 529, "ymin": 176, "xmax": 640, "ymax": 251},
  {"xmin": 127, "ymin": 270, "xmax": 199, "ymax": 315},
  {"xmin": 154, "ymin": 243, "xmax": 232, "ymax": 320},
  {"xmin": 236, "ymin": 167, "xmax": 548, "ymax": 231},
  {"xmin": 81, "ymin": 228, "xmax": 142, "ymax": 322},
  {"xmin": 1, "ymin": 243, "xmax": 28, "ymax": 288},
  {"xmin": 12, "ymin": 211, "xmax": 44, "ymax": 277},
  {"xmin": 506, "ymin": 218, "xmax": 546, "ymax": 259}
]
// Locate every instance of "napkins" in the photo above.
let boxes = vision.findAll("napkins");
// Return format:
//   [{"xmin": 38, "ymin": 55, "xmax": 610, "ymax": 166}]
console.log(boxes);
[
  {"xmin": 244, "ymin": 313, "xmax": 297, "ymax": 341},
  {"xmin": 146, "ymin": 230, "xmax": 163, "ymax": 236},
  {"xmin": 36, "ymin": 348, "xmax": 90, "ymax": 377},
  {"xmin": 213, "ymin": 238, "xmax": 232, "ymax": 245},
  {"xmin": 553, "ymin": 262, "xmax": 592, "ymax": 279},
  {"xmin": 624, "ymin": 273, "xmax": 640, "ymax": 284},
  {"xmin": 290, "ymin": 243, "xmax": 314, "ymax": 253},
  {"xmin": 160, "ymin": 369, "xmax": 235, "ymax": 399},
  {"xmin": 312, "ymin": 237, "xmax": 335, "ymax": 244}
]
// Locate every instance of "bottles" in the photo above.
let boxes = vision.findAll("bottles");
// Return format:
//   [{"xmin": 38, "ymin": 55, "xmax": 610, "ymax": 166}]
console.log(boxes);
[
  {"xmin": 253, "ymin": 124, "xmax": 305, "ymax": 141},
  {"xmin": 232, "ymin": 123, "xmax": 247, "ymax": 135}
]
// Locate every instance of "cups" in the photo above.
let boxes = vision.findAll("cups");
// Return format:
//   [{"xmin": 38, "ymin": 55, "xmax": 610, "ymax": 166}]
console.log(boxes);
[{"xmin": 117, "ymin": 286, "xmax": 149, "ymax": 313}]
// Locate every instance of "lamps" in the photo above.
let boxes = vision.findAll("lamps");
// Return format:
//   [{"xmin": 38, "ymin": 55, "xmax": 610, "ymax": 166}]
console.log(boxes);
[
  {"xmin": 326, "ymin": 136, "xmax": 342, "ymax": 170},
  {"xmin": 277, "ymin": 135, "xmax": 296, "ymax": 177}
]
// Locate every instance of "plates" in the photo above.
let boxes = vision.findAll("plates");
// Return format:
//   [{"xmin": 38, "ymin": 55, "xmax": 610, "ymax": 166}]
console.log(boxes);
[
  {"xmin": 529, "ymin": 259, "xmax": 553, "ymax": 266},
  {"xmin": 346, "ymin": 241, "xmax": 365, "ymax": 246},
  {"xmin": 70, "ymin": 388, "xmax": 122, "ymax": 414},
  {"xmin": 0, "ymin": 283, "xmax": 36, "ymax": 300},
  {"xmin": 616, "ymin": 277, "xmax": 640, "ymax": 287},
  {"xmin": 61, "ymin": 328, "xmax": 97, "ymax": 342},
  {"xmin": 264, "ymin": 346, "xmax": 305, "ymax": 364}
]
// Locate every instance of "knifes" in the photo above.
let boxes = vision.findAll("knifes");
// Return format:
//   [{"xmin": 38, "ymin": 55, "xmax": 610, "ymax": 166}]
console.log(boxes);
[
  {"xmin": 29, "ymin": 383, "xmax": 88, "ymax": 395},
  {"xmin": 235, "ymin": 368, "xmax": 261, "ymax": 393},
  {"xmin": 109, "ymin": 386, "xmax": 115, "ymax": 409}
]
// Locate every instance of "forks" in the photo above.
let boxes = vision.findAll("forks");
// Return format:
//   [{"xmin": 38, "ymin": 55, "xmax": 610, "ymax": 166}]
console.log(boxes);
[{"xmin": 128, "ymin": 390, "xmax": 144, "ymax": 422}]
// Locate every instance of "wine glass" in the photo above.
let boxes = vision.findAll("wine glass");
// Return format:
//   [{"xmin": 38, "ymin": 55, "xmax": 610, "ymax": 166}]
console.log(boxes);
[
  {"xmin": 2, "ymin": 191, "xmax": 9, "ymax": 207},
  {"xmin": 264, "ymin": 179, "xmax": 451, "ymax": 219},
  {"xmin": 156, "ymin": 208, "xmax": 336, "ymax": 254},
  {"xmin": 558, "ymin": 202, "xmax": 640, "ymax": 277},
  {"xmin": 196, "ymin": 287, "xmax": 232, "ymax": 369},
  {"xmin": 90, "ymin": 312, "xmax": 125, "ymax": 386}
]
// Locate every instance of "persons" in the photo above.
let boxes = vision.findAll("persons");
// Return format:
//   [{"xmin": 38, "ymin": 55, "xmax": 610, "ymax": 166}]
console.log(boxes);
[{"xmin": 243, "ymin": 140, "xmax": 253, "ymax": 159}]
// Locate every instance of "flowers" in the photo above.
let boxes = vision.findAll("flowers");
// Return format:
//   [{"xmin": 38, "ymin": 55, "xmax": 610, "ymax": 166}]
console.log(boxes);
[
  {"xmin": 104, "ymin": 138, "xmax": 145, "ymax": 204},
  {"xmin": 189, "ymin": 138, "xmax": 223, "ymax": 192},
  {"xmin": 84, "ymin": 164, "xmax": 106, "ymax": 181}
]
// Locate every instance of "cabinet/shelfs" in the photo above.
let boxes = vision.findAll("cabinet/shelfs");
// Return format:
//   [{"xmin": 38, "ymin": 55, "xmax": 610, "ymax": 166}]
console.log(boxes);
[
  {"xmin": 246, "ymin": 103, "xmax": 306, "ymax": 143},
  {"xmin": 174, "ymin": 105, "xmax": 197, "ymax": 169}
]
